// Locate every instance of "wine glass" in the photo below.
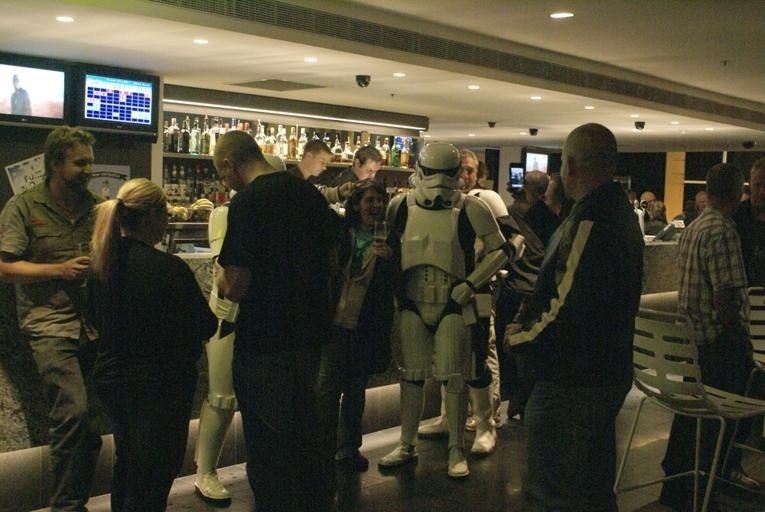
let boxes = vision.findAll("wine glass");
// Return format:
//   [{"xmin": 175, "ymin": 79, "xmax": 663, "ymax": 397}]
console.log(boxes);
[{"xmin": 77, "ymin": 241, "xmax": 90, "ymax": 286}]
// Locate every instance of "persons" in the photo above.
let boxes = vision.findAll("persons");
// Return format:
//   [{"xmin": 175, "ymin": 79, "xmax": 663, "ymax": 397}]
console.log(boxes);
[
  {"xmin": 2, "ymin": 125, "xmax": 113, "ymax": 510},
  {"xmin": 379, "ymin": 141, "xmax": 511, "ymax": 477},
  {"xmin": 194, "ymin": 129, "xmax": 394, "ymax": 512},
  {"xmin": 624, "ymin": 190, "xmax": 667, "ymax": 236},
  {"xmin": 83, "ymin": 177, "xmax": 218, "ymax": 512},
  {"xmin": 658, "ymin": 158, "xmax": 765, "ymax": 512},
  {"xmin": 496, "ymin": 124, "xmax": 645, "ymax": 510},
  {"xmin": 11, "ymin": 74, "xmax": 31, "ymax": 116}
]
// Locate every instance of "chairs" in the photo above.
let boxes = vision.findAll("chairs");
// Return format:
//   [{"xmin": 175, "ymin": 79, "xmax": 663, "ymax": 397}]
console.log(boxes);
[
  {"xmin": 744, "ymin": 287, "xmax": 765, "ymax": 396},
  {"xmin": 614, "ymin": 307, "xmax": 765, "ymax": 512}
]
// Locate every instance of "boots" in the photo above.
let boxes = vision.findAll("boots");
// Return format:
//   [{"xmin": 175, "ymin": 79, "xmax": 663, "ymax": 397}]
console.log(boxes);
[
  {"xmin": 378, "ymin": 373, "xmax": 502, "ymax": 480},
  {"xmin": 193, "ymin": 399, "xmax": 236, "ymax": 503}
]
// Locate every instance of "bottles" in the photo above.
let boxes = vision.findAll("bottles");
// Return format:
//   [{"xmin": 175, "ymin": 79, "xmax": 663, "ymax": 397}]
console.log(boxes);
[
  {"xmin": 163, "ymin": 113, "xmax": 417, "ymax": 207},
  {"xmin": 100, "ymin": 179, "xmax": 110, "ymax": 202}
]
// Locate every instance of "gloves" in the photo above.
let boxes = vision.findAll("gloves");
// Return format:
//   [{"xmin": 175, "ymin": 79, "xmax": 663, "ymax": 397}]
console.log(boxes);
[{"xmin": 450, "ymin": 282, "xmax": 475, "ymax": 312}]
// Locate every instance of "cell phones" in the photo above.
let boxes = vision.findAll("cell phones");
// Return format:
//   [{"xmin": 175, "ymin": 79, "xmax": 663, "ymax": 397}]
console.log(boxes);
[{"xmin": 509, "ymin": 162, "xmax": 525, "ymax": 193}]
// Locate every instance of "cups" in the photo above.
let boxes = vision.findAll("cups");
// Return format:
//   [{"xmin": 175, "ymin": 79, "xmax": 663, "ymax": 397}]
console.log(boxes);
[{"xmin": 374, "ymin": 220, "xmax": 387, "ymax": 248}]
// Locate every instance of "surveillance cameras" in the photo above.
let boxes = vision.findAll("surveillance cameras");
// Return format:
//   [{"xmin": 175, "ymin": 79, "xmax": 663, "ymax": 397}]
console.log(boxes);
[
  {"xmin": 529, "ymin": 129, "xmax": 538, "ymax": 135},
  {"xmin": 488, "ymin": 122, "xmax": 496, "ymax": 128},
  {"xmin": 356, "ymin": 75, "xmax": 370, "ymax": 86},
  {"xmin": 743, "ymin": 141, "xmax": 754, "ymax": 148},
  {"xmin": 635, "ymin": 122, "xmax": 645, "ymax": 129}
]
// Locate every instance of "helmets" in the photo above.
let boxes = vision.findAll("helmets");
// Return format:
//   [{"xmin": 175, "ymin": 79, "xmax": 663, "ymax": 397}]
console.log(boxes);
[{"xmin": 409, "ymin": 142, "xmax": 462, "ymax": 209}]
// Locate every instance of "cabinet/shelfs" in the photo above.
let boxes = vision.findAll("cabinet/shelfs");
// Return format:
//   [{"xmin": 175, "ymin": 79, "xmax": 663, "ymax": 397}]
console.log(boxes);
[{"xmin": 163, "ymin": 99, "xmax": 420, "ymax": 224}]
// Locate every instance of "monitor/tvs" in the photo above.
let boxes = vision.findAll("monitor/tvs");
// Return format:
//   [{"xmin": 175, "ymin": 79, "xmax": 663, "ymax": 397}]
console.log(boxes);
[
  {"xmin": 72, "ymin": 61, "xmax": 160, "ymax": 136},
  {"xmin": 0, "ymin": 51, "xmax": 72, "ymax": 129},
  {"xmin": 652, "ymin": 219, "xmax": 686, "ymax": 241},
  {"xmin": 521, "ymin": 148, "xmax": 551, "ymax": 176}
]
